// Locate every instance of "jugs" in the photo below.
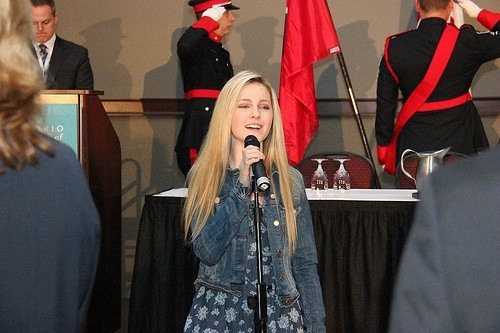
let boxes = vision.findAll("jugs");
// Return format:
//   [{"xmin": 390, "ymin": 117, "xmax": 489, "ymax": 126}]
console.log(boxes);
[{"xmin": 401, "ymin": 146, "xmax": 451, "ymax": 190}]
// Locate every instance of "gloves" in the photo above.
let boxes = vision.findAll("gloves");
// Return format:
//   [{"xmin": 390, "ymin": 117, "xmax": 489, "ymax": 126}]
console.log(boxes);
[
  {"xmin": 453, "ymin": 0, "xmax": 482, "ymax": 19},
  {"xmin": 382, "ymin": 165, "xmax": 386, "ymax": 173},
  {"xmin": 201, "ymin": 7, "xmax": 226, "ymax": 22}
]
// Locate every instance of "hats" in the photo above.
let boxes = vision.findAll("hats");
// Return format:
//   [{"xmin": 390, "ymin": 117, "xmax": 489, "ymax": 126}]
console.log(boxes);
[{"xmin": 188, "ymin": 0, "xmax": 240, "ymax": 13}]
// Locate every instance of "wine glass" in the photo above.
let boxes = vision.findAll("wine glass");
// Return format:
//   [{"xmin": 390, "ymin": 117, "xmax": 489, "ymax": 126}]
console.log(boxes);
[
  {"xmin": 333, "ymin": 159, "xmax": 350, "ymax": 190},
  {"xmin": 311, "ymin": 158, "xmax": 329, "ymax": 191}
]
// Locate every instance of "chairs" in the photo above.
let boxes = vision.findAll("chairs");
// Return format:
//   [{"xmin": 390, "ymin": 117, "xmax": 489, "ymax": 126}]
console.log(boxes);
[{"xmin": 295, "ymin": 152, "xmax": 469, "ymax": 190}]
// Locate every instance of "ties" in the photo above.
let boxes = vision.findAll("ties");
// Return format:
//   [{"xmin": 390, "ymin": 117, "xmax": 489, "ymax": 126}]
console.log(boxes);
[{"xmin": 39, "ymin": 44, "xmax": 48, "ymax": 66}]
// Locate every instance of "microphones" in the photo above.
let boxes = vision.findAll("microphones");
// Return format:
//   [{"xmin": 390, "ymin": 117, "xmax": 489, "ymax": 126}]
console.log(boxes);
[{"xmin": 244, "ymin": 135, "xmax": 271, "ymax": 192}]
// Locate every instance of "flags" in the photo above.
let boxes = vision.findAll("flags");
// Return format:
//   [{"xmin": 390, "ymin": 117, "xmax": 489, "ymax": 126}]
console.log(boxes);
[{"xmin": 276, "ymin": 0, "xmax": 341, "ymax": 170}]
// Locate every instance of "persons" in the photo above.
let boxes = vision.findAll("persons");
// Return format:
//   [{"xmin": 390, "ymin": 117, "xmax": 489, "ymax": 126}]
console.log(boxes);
[
  {"xmin": 26, "ymin": 1, "xmax": 94, "ymax": 88},
  {"xmin": 376, "ymin": 0, "xmax": 499, "ymax": 175},
  {"xmin": 174, "ymin": 0, "xmax": 241, "ymax": 184},
  {"xmin": 181, "ymin": 69, "xmax": 328, "ymax": 333},
  {"xmin": 387, "ymin": 139, "xmax": 500, "ymax": 333},
  {"xmin": 0, "ymin": 0, "xmax": 103, "ymax": 332}
]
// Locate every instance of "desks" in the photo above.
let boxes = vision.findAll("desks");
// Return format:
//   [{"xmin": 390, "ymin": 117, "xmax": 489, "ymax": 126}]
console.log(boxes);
[{"xmin": 144, "ymin": 187, "xmax": 421, "ymax": 333}]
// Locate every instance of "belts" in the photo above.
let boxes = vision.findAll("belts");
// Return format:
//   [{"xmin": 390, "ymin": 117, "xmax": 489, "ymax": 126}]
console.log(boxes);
[
  {"xmin": 416, "ymin": 92, "xmax": 473, "ymax": 112},
  {"xmin": 184, "ymin": 89, "xmax": 220, "ymax": 98}
]
